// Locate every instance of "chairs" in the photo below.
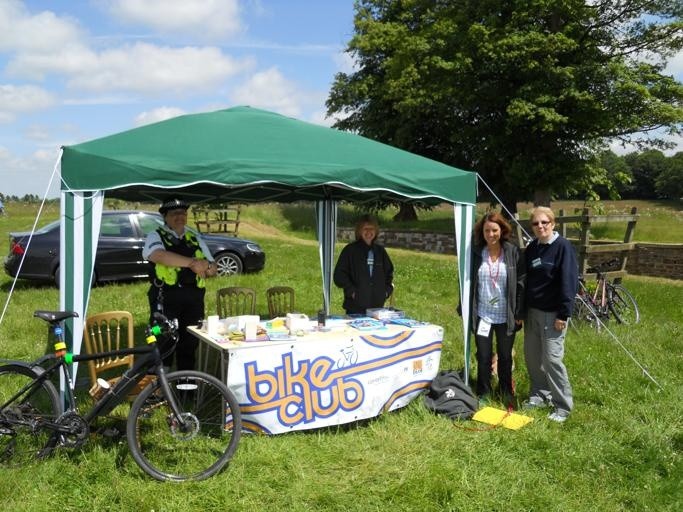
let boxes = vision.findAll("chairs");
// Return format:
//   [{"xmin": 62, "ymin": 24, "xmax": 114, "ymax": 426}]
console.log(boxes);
[
  {"xmin": 215, "ymin": 287, "xmax": 256, "ymax": 320},
  {"xmin": 82, "ymin": 310, "xmax": 174, "ymax": 455},
  {"xmin": 267, "ymin": 286, "xmax": 294, "ymax": 319}
]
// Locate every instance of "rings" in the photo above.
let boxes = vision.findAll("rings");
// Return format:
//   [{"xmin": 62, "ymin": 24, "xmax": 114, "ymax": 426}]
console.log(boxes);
[{"xmin": 562, "ymin": 326, "xmax": 564, "ymax": 327}]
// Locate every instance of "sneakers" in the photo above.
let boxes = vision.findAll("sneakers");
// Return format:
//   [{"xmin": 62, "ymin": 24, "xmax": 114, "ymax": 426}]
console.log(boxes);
[
  {"xmin": 549, "ymin": 410, "xmax": 567, "ymax": 424},
  {"xmin": 524, "ymin": 394, "xmax": 546, "ymax": 411}
]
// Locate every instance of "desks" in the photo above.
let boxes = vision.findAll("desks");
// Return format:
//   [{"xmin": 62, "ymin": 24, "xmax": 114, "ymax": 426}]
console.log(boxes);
[{"xmin": 185, "ymin": 315, "xmax": 443, "ymax": 436}]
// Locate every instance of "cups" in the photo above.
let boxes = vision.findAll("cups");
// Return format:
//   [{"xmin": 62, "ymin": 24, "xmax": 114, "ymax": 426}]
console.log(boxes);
[
  {"xmin": 237, "ymin": 311, "xmax": 259, "ymax": 342},
  {"xmin": 206, "ymin": 314, "xmax": 218, "ymax": 338},
  {"xmin": 88, "ymin": 378, "xmax": 110, "ymax": 401}
]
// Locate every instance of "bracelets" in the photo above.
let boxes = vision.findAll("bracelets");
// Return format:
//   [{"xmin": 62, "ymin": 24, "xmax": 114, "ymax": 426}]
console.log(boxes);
[{"xmin": 208, "ymin": 263, "xmax": 213, "ymax": 270}]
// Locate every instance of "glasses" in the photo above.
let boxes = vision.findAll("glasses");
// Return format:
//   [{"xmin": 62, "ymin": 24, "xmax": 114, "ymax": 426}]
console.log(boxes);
[{"xmin": 531, "ymin": 220, "xmax": 551, "ymax": 226}]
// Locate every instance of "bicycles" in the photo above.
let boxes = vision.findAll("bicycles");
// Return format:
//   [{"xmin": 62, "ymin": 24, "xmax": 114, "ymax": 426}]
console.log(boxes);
[
  {"xmin": 0, "ymin": 310, "xmax": 240, "ymax": 481},
  {"xmin": 569, "ymin": 258, "xmax": 640, "ymax": 339}
]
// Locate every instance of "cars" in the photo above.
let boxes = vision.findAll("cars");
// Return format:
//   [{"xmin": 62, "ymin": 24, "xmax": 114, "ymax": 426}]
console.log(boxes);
[{"xmin": 3, "ymin": 209, "xmax": 264, "ymax": 290}]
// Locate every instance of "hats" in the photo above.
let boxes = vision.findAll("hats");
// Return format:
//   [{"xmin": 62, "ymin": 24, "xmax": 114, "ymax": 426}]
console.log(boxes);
[{"xmin": 158, "ymin": 195, "xmax": 189, "ymax": 213}]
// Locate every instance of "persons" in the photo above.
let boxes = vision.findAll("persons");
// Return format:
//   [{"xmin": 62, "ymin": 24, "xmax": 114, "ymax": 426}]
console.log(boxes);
[
  {"xmin": 521, "ymin": 205, "xmax": 580, "ymax": 425},
  {"xmin": 333, "ymin": 215, "xmax": 395, "ymax": 313},
  {"xmin": 456, "ymin": 215, "xmax": 526, "ymax": 407},
  {"xmin": 142, "ymin": 200, "xmax": 218, "ymax": 407}
]
